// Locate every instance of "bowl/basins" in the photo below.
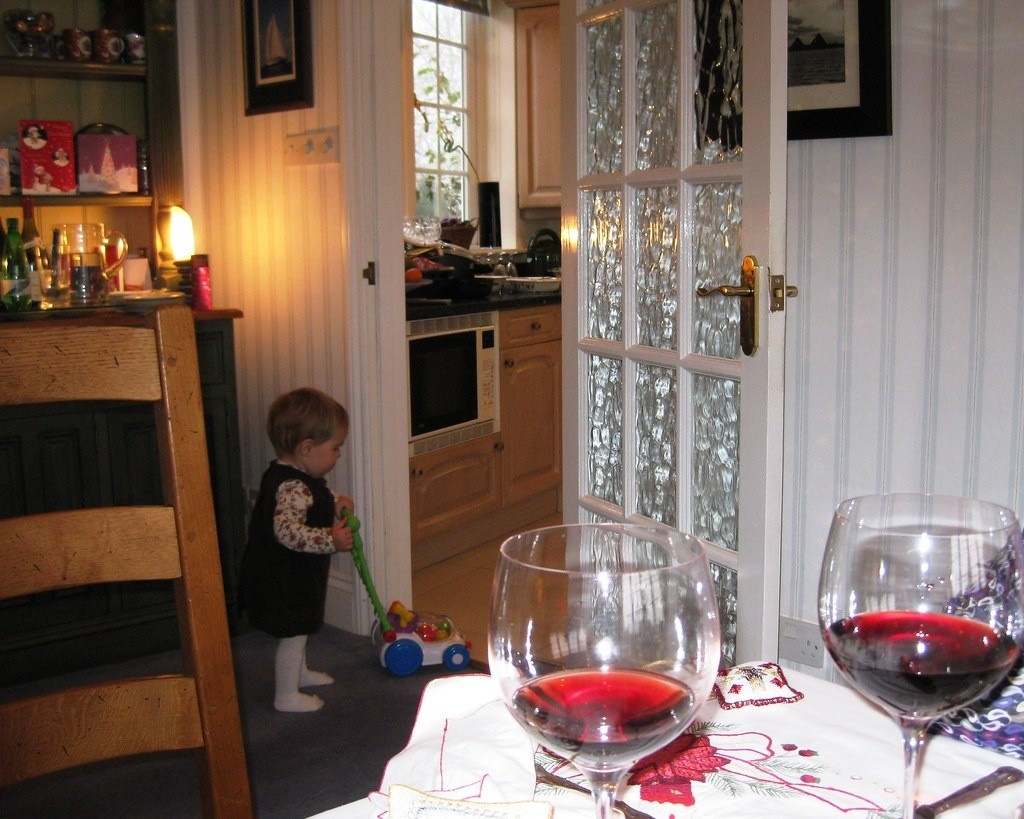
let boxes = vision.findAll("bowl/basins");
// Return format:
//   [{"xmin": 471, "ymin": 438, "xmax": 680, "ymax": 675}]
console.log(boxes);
[{"xmin": 475, "ymin": 274, "xmax": 562, "ymax": 293}]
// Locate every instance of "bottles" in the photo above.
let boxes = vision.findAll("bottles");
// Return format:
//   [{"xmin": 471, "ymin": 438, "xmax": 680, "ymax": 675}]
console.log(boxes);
[
  {"xmin": 16, "ymin": 198, "xmax": 53, "ymax": 303},
  {"xmin": 49, "ymin": 226, "xmax": 71, "ymax": 293},
  {"xmin": 1, "ymin": 215, "xmax": 33, "ymax": 312}
]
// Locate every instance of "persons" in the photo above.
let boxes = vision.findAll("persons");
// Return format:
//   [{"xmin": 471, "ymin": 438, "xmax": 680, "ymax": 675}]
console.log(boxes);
[{"xmin": 240, "ymin": 387, "xmax": 355, "ymax": 712}]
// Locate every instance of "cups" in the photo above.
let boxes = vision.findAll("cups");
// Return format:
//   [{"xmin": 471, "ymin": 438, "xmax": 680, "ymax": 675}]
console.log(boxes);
[
  {"xmin": 125, "ymin": 32, "xmax": 146, "ymax": 64},
  {"xmin": 95, "ymin": 27, "xmax": 122, "ymax": 63},
  {"xmin": 65, "ymin": 222, "xmax": 131, "ymax": 304},
  {"xmin": 62, "ymin": 28, "xmax": 92, "ymax": 61},
  {"xmin": 137, "ymin": 139, "xmax": 149, "ymax": 194},
  {"xmin": 403, "ymin": 216, "xmax": 443, "ymax": 241}
]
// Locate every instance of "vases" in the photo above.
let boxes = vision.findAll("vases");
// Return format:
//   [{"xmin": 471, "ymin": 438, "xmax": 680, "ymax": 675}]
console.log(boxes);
[{"xmin": 441, "ymin": 224, "xmax": 476, "ymax": 250}]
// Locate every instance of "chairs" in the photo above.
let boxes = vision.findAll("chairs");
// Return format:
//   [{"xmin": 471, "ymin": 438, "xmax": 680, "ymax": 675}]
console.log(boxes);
[{"xmin": 2, "ymin": 300, "xmax": 268, "ymax": 818}]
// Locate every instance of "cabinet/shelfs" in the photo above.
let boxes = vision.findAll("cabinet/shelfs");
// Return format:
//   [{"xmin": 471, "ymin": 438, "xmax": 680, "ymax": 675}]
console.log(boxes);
[
  {"xmin": 513, "ymin": 1, "xmax": 565, "ymax": 223},
  {"xmin": 1, "ymin": 1, "xmax": 251, "ymax": 703}
]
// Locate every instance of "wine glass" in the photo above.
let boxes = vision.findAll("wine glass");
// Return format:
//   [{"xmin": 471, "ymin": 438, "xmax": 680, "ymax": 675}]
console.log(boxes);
[
  {"xmin": 486, "ymin": 520, "xmax": 722, "ymax": 818},
  {"xmin": 815, "ymin": 492, "xmax": 1024, "ymax": 819}
]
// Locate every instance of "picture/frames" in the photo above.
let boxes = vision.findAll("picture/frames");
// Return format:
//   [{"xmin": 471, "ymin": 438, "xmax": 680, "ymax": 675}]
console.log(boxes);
[
  {"xmin": 692, "ymin": 0, "xmax": 897, "ymax": 154},
  {"xmin": 230, "ymin": 0, "xmax": 317, "ymax": 118}
]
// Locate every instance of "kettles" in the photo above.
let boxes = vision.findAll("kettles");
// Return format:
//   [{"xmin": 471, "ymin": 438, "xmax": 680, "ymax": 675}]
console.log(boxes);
[{"xmin": 523, "ymin": 230, "xmax": 561, "ymax": 270}]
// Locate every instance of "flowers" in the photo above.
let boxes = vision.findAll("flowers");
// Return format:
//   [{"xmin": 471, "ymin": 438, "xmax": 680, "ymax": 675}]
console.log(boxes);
[{"xmin": 435, "ymin": 211, "xmax": 477, "ymax": 230}]
